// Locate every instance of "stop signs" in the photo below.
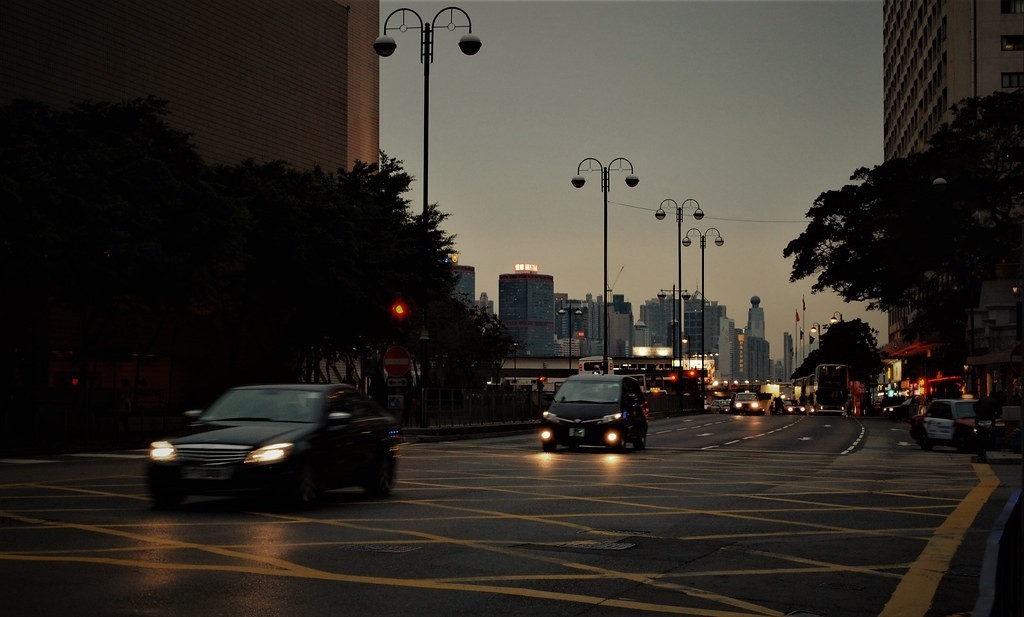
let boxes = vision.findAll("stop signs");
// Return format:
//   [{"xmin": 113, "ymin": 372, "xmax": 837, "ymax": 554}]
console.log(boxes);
[{"xmin": 384, "ymin": 346, "xmax": 411, "ymax": 378}]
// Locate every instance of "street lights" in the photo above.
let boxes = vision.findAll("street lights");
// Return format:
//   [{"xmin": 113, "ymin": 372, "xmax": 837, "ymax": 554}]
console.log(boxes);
[
  {"xmin": 811, "ymin": 322, "xmax": 820, "ymax": 350},
  {"xmin": 570, "ymin": 156, "xmax": 639, "ymax": 372},
  {"xmin": 682, "ymin": 226, "xmax": 723, "ymax": 405},
  {"xmin": 831, "ymin": 310, "xmax": 843, "ymax": 325},
  {"xmin": 373, "ymin": 4, "xmax": 483, "ymax": 215},
  {"xmin": 656, "ymin": 285, "xmax": 691, "ymax": 375},
  {"xmin": 653, "ymin": 198, "xmax": 704, "ymax": 414}
]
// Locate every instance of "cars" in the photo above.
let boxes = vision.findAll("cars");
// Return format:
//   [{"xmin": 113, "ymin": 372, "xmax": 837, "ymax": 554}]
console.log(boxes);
[
  {"xmin": 781, "ymin": 399, "xmax": 806, "ymax": 415},
  {"xmin": 909, "ymin": 398, "xmax": 1005, "ymax": 454},
  {"xmin": 709, "ymin": 399, "xmax": 729, "ymax": 413},
  {"xmin": 540, "ymin": 372, "xmax": 648, "ymax": 453},
  {"xmin": 143, "ymin": 381, "xmax": 399, "ymax": 514},
  {"xmin": 734, "ymin": 389, "xmax": 762, "ymax": 414},
  {"xmin": 883, "ymin": 398, "xmax": 922, "ymax": 421}
]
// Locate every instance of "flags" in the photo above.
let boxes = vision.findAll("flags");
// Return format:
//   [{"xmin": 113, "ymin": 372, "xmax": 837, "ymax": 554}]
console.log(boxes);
[{"xmin": 796, "ymin": 297, "xmax": 816, "ymax": 346}]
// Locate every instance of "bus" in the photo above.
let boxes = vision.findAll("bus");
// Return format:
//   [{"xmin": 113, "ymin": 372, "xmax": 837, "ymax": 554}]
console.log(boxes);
[{"xmin": 812, "ymin": 362, "xmax": 850, "ymax": 416}]
